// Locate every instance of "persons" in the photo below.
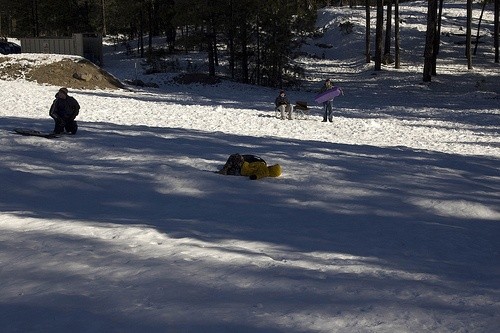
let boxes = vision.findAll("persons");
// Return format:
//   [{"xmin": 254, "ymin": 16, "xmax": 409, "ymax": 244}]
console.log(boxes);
[
  {"xmin": 274, "ymin": 91, "xmax": 293, "ymax": 120},
  {"xmin": 49, "ymin": 88, "xmax": 81, "ymax": 136},
  {"xmin": 316, "ymin": 78, "xmax": 344, "ymax": 122},
  {"xmin": 221, "ymin": 154, "xmax": 282, "ymax": 180}
]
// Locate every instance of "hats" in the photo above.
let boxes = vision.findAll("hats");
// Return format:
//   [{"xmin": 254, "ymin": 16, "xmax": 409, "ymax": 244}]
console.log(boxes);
[{"xmin": 60, "ymin": 88, "xmax": 68, "ymax": 95}]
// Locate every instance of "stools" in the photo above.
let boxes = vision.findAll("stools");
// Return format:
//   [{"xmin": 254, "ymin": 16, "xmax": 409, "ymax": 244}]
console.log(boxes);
[
  {"xmin": 275, "ymin": 106, "xmax": 289, "ymax": 112},
  {"xmin": 54, "ymin": 120, "xmax": 78, "ymax": 135}
]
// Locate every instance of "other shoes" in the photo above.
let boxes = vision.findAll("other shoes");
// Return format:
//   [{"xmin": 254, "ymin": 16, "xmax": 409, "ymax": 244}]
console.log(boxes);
[
  {"xmin": 288, "ymin": 117, "xmax": 293, "ymax": 120},
  {"xmin": 219, "ymin": 170, "xmax": 228, "ymax": 175},
  {"xmin": 321, "ymin": 120, "xmax": 327, "ymax": 122},
  {"xmin": 329, "ymin": 120, "xmax": 333, "ymax": 122},
  {"xmin": 282, "ymin": 117, "xmax": 285, "ymax": 120}
]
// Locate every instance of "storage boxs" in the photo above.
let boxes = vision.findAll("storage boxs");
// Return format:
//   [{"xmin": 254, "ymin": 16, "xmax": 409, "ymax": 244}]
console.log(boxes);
[
  {"xmin": 296, "ymin": 100, "xmax": 307, "ymax": 110},
  {"xmin": 313, "ymin": 86, "xmax": 342, "ymax": 105}
]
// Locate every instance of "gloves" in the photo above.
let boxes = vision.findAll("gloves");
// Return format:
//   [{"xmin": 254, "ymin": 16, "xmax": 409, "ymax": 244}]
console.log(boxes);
[{"xmin": 250, "ymin": 175, "xmax": 257, "ymax": 180}]
